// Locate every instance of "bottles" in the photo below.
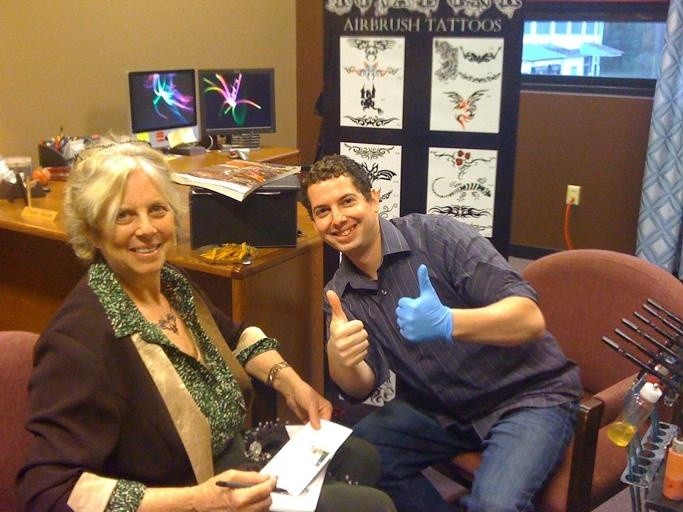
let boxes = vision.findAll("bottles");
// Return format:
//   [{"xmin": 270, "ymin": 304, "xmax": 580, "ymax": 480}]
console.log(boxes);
[
  {"xmin": 661, "ymin": 432, "xmax": 683, "ymax": 501},
  {"xmin": 606, "ymin": 381, "xmax": 663, "ymax": 446}
]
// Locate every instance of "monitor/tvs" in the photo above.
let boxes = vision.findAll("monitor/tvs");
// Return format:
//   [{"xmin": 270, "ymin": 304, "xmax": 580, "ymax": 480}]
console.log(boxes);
[
  {"xmin": 198, "ymin": 67, "xmax": 276, "ymax": 135},
  {"xmin": 125, "ymin": 69, "xmax": 200, "ymax": 153}
]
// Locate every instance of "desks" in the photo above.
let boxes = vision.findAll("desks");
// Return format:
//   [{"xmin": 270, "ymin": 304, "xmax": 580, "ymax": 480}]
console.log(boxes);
[{"xmin": 1, "ymin": 144, "xmax": 329, "ymax": 439}]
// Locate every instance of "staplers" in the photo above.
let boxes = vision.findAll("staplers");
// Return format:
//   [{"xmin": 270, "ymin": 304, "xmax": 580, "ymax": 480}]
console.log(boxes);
[{"xmin": 48, "ymin": 166, "xmax": 70, "ymax": 181}]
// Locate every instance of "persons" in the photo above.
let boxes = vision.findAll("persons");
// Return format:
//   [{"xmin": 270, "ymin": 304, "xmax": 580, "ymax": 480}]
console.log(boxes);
[
  {"xmin": 11, "ymin": 129, "xmax": 399, "ymax": 512},
  {"xmin": 300, "ymin": 151, "xmax": 587, "ymax": 512}
]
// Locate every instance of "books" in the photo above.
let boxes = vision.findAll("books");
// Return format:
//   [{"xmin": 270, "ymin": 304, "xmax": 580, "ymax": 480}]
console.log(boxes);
[{"xmin": 178, "ymin": 157, "xmax": 302, "ymax": 203}]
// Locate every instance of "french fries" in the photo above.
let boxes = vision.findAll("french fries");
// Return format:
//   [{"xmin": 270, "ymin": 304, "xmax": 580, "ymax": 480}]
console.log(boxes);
[{"xmin": 201, "ymin": 241, "xmax": 255, "ymax": 261}]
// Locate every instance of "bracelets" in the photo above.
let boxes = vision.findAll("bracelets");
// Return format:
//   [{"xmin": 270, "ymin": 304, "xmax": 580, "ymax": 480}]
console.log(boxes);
[{"xmin": 265, "ymin": 361, "xmax": 294, "ymax": 389}]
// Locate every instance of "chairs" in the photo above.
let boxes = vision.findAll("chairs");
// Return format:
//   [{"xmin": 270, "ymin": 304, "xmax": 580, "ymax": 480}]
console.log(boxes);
[
  {"xmin": 430, "ymin": 247, "xmax": 683, "ymax": 512},
  {"xmin": 0, "ymin": 329, "xmax": 40, "ymax": 512}
]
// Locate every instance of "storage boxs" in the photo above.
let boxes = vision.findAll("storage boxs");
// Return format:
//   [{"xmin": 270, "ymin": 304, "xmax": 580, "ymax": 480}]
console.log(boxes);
[{"xmin": 189, "ymin": 174, "xmax": 300, "ymax": 251}]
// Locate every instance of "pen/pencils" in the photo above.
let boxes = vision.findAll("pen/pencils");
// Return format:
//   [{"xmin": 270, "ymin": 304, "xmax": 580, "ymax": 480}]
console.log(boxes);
[
  {"xmin": 216, "ymin": 481, "xmax": 288, "ymax": 494},
  {"xmin": 40, "ymin": 126, "xmax": 85, "ymax": 153}
]
